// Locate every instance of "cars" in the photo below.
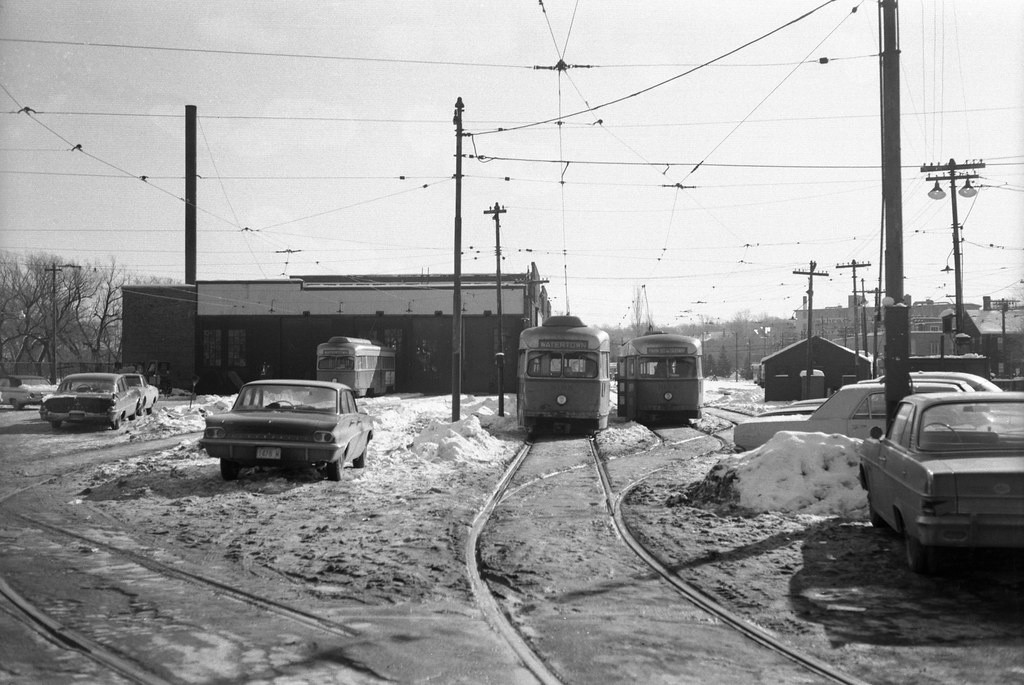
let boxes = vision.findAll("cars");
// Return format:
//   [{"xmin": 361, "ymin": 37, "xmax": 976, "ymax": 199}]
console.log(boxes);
[
  {"xmin": 0, "ymin": 375, "xmax": 58, "ymax": 409},
  {"xmin": 859, "ymin": 389, "xmax": 1024, "ymax": 572},
  {"xmin": 41, "ymin": 374, "xmax": 141, "ymax": 429},
  {"xmin": 733, "ymin": 381, "xmax": 973, "ymax": 452},
  {"xmin": 201, "ymin": 379, "xmax": 373, "ymax": 481},
  {"xmin": 760, "ymin": 371, "xmax": 1002, "ymax": 415},
  {"xmin": 125, "ymin": 374, "xmax": 159, "ymax": 416}
]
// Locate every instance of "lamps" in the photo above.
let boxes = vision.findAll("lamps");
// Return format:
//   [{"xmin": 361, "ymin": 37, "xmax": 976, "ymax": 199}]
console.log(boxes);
[
  {"xmin": 435, "ymin": 310, "xmax": 442, "ymax": 318},
  {"xmin": 268, "ymin": 300, "xmax": 276, "ymax": 313},
  {"xmin": 461, "ymin": 302, "xmax": 467, "ymax": 313},
  {"xmin": 406, "ymin": 302, "xmax": 413, "ymax": 313},
  {"xmin": 483, "ymin": 310, "xmax": 491, "ymax": 316},
  {"xmin": 303, "ymin": 311, "xmax": 310, "ymax": 317},
  {"xmin": 376, "ymin": 311, "xmax": 384, "ymax": 317},
  {"xmin": 336, "ymin": 303, "xmax": 343, "ymax": 313}
]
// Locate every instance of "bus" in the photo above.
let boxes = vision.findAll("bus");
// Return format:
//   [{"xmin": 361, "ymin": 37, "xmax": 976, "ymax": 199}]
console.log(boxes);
[
  {"xmin": 518, "ymin": 315, "xmax": 611, "ymax": 433},
  {"xmin": 618, "ymin": 328, "xmax": 703, "ymax": 425},
  {"xmin": 316, "ymin": 337, "xmax": 397, "ymax": 397}
]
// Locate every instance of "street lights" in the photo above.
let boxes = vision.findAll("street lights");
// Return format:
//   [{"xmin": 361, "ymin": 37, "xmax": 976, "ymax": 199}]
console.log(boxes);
[{"xmin": 922, "ymin": 160, "xmax": 987, "ymax": 353}]
